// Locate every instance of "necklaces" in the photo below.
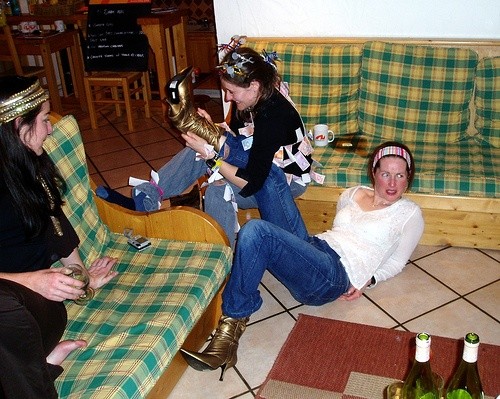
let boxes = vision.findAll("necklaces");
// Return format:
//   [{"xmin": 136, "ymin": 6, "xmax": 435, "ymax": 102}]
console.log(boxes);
[{"xmin": 370, "ymin": 199, "xmax": 389, "ymax": 207}]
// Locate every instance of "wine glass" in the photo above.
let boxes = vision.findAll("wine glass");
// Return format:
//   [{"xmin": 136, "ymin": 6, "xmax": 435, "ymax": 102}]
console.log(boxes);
[{"xmin": 60, "ymin": 263, "xmax": 95, "ymax": 305}]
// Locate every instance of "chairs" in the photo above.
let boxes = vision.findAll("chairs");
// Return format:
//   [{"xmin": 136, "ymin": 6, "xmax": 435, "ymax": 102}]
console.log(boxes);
[{"xmin": 82, "ymin": 5, "xmax": 155, "ymax": 133}]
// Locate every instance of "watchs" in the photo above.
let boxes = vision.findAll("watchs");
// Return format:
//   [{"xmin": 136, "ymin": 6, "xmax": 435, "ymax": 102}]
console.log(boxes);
[{"xmin": 206, "ymin": 154, "xmax": 220, "ymax": 168}]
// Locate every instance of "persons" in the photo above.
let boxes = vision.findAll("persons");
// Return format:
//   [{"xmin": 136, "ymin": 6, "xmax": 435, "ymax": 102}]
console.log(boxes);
[
  {"xmin": 94, "ymin": 47, "xmax": 313, "ymax": 251},
  {"xmin": 0, "ymin": 75, "xmax": 119, "ymax": 399},
  {"xmin": 165, "ymin": 64, "xmax": 425, "ymax": 381}
]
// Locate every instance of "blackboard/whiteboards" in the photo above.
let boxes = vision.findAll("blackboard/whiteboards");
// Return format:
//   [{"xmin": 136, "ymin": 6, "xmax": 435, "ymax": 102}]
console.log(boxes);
[{"xmin": 85, "ymin": 3, "xmax": 152, "ymax": 72}]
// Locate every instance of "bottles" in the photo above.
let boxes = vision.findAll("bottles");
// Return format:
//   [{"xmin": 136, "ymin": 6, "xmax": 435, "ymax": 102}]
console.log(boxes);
[
  {"xmin": 5, "ymin": 0, "xmax": 20, "ymax": 16},
  {"xmin": 445, "ymin": 332, "xmax": 484, "ymax": 399},
  {"xmin": 401, "ymin": 332, "xmax": 439, "ymax": 399}
]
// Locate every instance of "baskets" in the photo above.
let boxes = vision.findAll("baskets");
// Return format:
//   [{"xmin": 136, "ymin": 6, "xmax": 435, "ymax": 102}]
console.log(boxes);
[{"xmin": 40, "ymin": 4, "xmax": 75, "ymax": 17}]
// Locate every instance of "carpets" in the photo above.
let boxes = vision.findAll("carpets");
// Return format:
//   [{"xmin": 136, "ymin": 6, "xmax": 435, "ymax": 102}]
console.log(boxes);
[{"xmin": 252, "ymin": 314, "xmax": 500, "ymax": 399}]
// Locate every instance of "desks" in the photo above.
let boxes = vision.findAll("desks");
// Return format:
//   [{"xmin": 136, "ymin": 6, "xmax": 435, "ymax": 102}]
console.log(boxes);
[
  {"xmin": 13, "ymin": 30, "xmax": 88, "ymax": 117},
  {"xmin": 5, "ymin": 3, "xmax": 188, "ymax": 118}
]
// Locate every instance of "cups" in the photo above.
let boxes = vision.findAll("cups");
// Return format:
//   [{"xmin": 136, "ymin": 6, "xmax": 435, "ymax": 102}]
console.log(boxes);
[
  {"xmin": 387, "ymin": 381, "xmax": 404, "ymax": 399},
  {"xmin": 313, "ymin": 123, "xmax": 334, "ymax": 147},
  {"xmin": 18, "ymin": 22, "xmax": 29, "ymax": 33},
  {"xmin": 54, "ymin": 20, "xmax": 64, "ymax": 32},
  {"xmin": 29, "ymin": 22, "xmax": 39, "ymax": 33},
  {"xmin": 432, "ymin": 370, "xmax": 445, "ymax": 399}
]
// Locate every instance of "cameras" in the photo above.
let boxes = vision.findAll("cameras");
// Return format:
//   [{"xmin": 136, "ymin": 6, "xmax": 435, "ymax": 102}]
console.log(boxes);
[{"xmin": 128, "ymin": 235, "xmax": 151, "ymax": 251}]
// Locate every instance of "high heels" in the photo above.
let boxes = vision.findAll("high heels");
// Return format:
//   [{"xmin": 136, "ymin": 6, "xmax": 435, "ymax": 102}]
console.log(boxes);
[
  {"xmin": 162, "ymin": 65, "xmax": 227, "ymax": 153},
  {"xmin": 179, "ymin": 315, "xmax": 250, "ymax": 382}
]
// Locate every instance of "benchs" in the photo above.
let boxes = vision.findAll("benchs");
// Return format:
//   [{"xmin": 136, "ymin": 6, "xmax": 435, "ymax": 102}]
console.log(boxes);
[
  {"xmin": 38, "ymin": 110, "xmax": 233, "ymax": 399},
  {"xmin": 200, "ymin": 35, "xmax": 499, "ymax": 251}
]
